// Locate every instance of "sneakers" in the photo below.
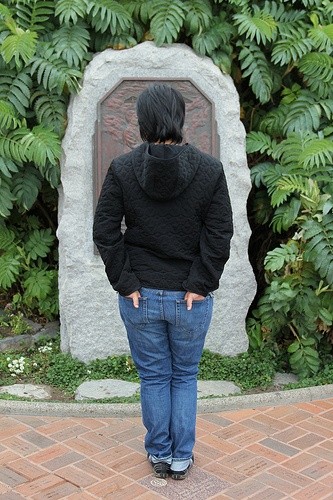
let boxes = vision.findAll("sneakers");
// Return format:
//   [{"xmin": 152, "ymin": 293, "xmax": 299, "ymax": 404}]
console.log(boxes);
[{"xmin": 142, "ymin": 455, "xmax": 196, "ymax": 480}]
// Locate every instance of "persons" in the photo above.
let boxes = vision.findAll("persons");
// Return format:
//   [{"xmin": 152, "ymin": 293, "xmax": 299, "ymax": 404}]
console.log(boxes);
[{"xmin": 92, "ymin": 84, "xmax": 234, "ymax": 480}]
